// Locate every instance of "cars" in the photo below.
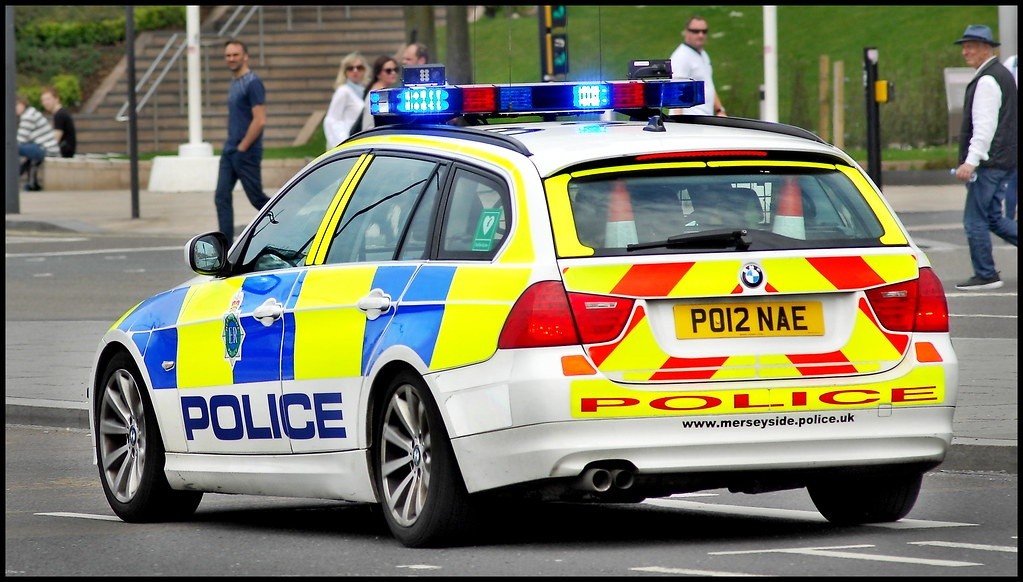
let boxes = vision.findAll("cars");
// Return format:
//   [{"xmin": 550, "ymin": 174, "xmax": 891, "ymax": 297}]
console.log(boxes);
[{"xmin": 88, "ymin": 56, "xmax": 961, "ymax": 551}]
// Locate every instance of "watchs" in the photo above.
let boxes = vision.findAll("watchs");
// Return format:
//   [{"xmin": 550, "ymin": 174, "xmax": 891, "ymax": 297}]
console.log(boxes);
[{"xmin": 716, "ymin": 107, "xmax": 725, "ymax": 112}]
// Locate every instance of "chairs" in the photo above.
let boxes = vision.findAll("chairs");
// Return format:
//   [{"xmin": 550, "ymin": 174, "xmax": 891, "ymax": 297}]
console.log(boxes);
[
  {"xmin": 575, "ymin": 184, "xmax": 686, "ymax": 249},
  {"xmin": 395, "ymin": 178, "xmax": 486, "ymax": 245},
  {"xmin": 693, "ymin": 187, "xmax": 765, "ymax": 229}
]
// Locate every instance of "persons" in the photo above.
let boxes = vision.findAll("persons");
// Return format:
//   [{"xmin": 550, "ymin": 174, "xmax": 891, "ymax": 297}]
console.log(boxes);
[
  {"xmin": 954, "ymin": 25, "xmax": 1018, "ymax": 290},
  {"xmin": 668, "ymin": 17, "xmax": 727, "ymax": 117},
  {"xmin": 323, "ymin": 53, "xmax": 372, "ymax": 151},
  {"xmin": 402, "ymin": 42, "xmax": 460, "ymax": 124},
  {"xmin": 360, "ymin": 56, "xmax": 400, "ymax": 131},
  {"xmin": 214, "ymin": 41, "xmax": 273, "ymax": 258},
  {"xmin": 1003, "ymin": 56, "xmax": 1018, "ymax": 220},
  {"xmin": 24, "ymin": 86, "xmax": 76, "ymax": 191},
  {"xmin": 14, "ymin": 98, "xmax": 61, "ymax": 176}
]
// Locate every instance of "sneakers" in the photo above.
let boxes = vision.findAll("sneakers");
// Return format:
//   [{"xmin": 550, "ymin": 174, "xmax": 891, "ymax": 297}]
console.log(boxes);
[{"xmin": 955, "ymin": 270, "xmax": 1004, "ymax": 291}]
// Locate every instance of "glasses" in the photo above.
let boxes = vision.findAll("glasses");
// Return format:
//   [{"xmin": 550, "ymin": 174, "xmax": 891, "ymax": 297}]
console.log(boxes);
[
  {"xmin": 346, "ymin": 65, "xmax": 364, "ymax": 71},
  {"xmin": 382, "ymin": 67, "xmax": 399, "ymax": 74},
  {"xmin": 688, "ymin": 28, "xmax": 708, "ymax": 33}
]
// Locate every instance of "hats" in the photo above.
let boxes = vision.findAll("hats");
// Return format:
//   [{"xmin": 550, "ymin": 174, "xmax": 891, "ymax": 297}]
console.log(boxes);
[{"xmin": 954, "ymin": 24, "xmax": 1001, "ymax": 47}]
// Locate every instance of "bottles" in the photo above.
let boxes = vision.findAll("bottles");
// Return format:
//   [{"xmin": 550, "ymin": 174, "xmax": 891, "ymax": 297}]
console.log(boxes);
[{"xmin": 950, "ymin": 168, "xmax": 978, "ymax": 183}]
